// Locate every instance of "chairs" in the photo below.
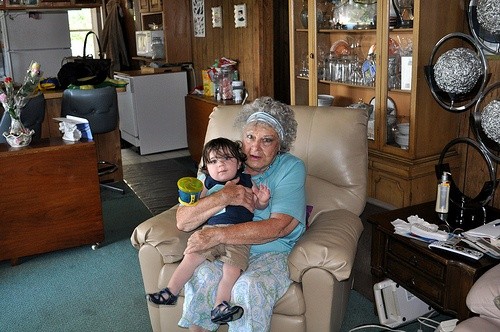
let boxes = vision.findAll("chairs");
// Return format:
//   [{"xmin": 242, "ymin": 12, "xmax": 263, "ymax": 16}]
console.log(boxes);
[
  {"xmin": 0, "ymin": 91, "xmax": 46, "ymax": 146},
  {"xmin": 61, "ymin": 88, "xmax": 124, "ymax": 193}
]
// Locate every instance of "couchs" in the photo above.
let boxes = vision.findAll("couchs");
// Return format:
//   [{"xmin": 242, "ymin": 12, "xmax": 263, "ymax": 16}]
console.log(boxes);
[{"xmin": 130, "ymin": 105, "xmax": 371, "ymax": 332}]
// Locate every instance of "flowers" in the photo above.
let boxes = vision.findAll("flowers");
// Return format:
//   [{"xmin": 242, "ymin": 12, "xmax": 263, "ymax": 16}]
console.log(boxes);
[{"xmin": 0, "ymin": 61, "xmax": 43, "ymax": 119}]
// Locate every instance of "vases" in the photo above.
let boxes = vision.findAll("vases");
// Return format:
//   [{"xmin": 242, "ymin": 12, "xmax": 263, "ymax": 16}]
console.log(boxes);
[{"xmin": 2, "ymin": 118, "xmax": 35, "ymax": 147}]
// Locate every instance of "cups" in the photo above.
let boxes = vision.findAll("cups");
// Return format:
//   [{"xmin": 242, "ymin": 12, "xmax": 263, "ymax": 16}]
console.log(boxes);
[
  {"xmin": 177, "ymin": 177, "xmax": 203, "ymax": 207},
  {"xmin": 231, "ymin": 81, "xmax": 243, "ymax": 101},
  {"xmin": 299, "ymin": 46, "xmax": 365, "ymax": 84}
]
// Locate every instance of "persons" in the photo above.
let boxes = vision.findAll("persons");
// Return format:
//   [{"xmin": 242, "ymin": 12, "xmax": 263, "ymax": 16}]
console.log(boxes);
[
  {"xmin": 144, "ymin": 136, "xmax": 271, "ymax": 323},
  {"xmin": 237, "ymin": 10, "xmax": 244, "ymax": 21},
  {"xmin": 176, "ymin": 96, "xmax": 307, "ymax": 332}
]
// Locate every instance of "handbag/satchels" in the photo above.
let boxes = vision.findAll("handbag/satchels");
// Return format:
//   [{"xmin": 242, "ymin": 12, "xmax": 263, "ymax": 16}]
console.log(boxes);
[{"xmin": 74, "ymin": 32, "xmax": 111, "ymax": 89}]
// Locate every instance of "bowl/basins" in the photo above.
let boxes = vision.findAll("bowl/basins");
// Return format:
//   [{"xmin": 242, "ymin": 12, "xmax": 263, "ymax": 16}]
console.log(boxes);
[
  {"xmin": 317, "ymin": 95, "xmax": 336, "ymax": 106},
  {"xmin": 395, "ymin": 123, "xmax": 409, "ymax": 150}
]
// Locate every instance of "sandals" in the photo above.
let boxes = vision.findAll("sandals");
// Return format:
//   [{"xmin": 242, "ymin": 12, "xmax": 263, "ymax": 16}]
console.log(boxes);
[
  {"xmin": 146, "ymin": 287, "xmax": 177, "ymax": 306},
  {"xmin": 210, "ymin": 300, "xmax": 245, "ymax": 324}
]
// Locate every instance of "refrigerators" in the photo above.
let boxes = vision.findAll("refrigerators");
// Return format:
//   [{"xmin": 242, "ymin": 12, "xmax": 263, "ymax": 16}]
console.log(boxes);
[{"xmin": 0, "ymin": 9, "xmax": 72, "ymax": 85}]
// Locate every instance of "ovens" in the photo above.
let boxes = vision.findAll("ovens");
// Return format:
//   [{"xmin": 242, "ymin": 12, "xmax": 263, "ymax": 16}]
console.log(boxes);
[{"xmin": 113, "ymin": 71, "xmax": 188, "ymax": 155}]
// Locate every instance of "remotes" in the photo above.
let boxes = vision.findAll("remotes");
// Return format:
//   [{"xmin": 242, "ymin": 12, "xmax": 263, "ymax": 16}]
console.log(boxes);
[
  {"xmin": 410, "ymin": 223, "xmax": 449, "ymax": 241},
  {"xmin": 428, "ymin": 240, "xmax": 484, "ymax": 261}
]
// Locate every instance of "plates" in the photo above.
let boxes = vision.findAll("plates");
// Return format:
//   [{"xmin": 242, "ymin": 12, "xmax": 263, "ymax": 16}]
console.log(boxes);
[{"xmin": 369, "ymin": 96, "xmax": 397, "ymax": 126}]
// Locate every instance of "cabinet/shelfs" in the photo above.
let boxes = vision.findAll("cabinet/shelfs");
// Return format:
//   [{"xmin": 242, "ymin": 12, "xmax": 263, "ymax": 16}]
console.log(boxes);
[
  {"xmin": 185, "ymin": 93, "xmax": 248, "ymax": 165},
  {"xmin": 114, "ymin": 69, "xmax": 188, "ymax": 156},
  {"xmin": 138, "ymin": 0, "xmax": 192, "ymax": 63},
  {"xmin": 369, "ymin": 198, "xmax": 500, "ymax": 320},
  {"xmin": 288, "ymin": 0, "xmax": 459, "ymax": 208}
]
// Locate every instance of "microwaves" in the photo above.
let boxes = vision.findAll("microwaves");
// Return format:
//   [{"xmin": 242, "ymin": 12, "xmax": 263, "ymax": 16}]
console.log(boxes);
[{"xmin": 135, "ymin": 30, "xmax": 165, "ymax": 58}]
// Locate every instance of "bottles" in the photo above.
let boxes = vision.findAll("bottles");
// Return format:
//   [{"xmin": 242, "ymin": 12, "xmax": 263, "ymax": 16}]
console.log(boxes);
[
  {"xmin": 222, "ymin": 67, "xmax": 232, "ymax": 99},
  {"xmin": 349, "ymin": 99, "xmax": 374, "ymax": 120},
  {"xmin": 214, "ymin": 68, "xmax": 222, "ymax": 100},
  {"xmin": 150, "ymin": 37, "xmax": 164, "ymax": 60}
]
